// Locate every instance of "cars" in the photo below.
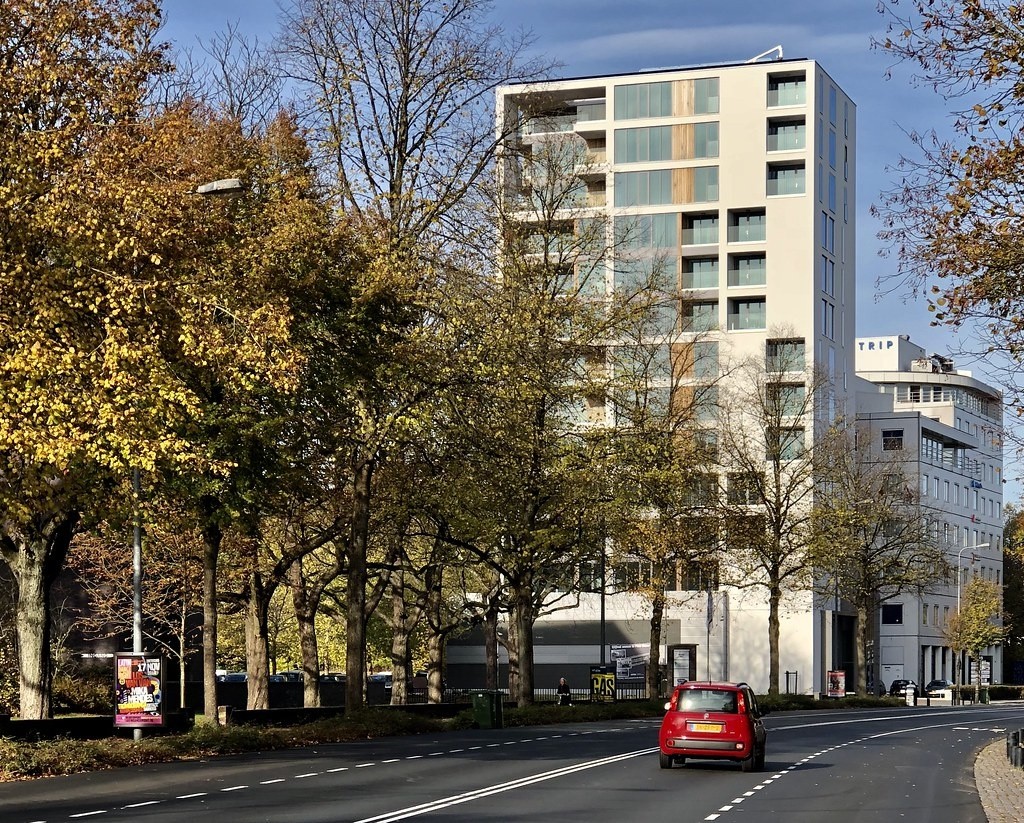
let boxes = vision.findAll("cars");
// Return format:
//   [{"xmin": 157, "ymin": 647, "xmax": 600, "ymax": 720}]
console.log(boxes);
[
  {"xmin": 269, "ymin": 675, "xmax": 288, "ymax": 682},
  {"xmin": 280, "ymin": 671, "xmax": 304, "ymax": 683},
  {"xmin": 867, "ymin": 680, "xmax": 887, "ymax": 698},
  {"xmin": 224, "ymin": 673, "xmax": 247, "ymax": 681},
  {"xmin": 926, "ymin": 680, "xmax": 954, "ymax": 698},
  {"xmin": 890, "ymin": 679, "xmax": 919, "ymax": 699}
]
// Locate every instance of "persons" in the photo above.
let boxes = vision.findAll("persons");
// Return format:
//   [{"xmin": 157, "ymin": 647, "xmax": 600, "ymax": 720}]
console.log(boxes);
[{"xmin": 555, "ymin": 678, "xmax": 571, "ymax": 706}]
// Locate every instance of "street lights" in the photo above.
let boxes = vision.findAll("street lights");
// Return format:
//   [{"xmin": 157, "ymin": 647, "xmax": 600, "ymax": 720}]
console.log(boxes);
[
  {"xmin": 957, "ymin": 543, "xmax": 991, "ymax": 706},
  {"xmin": 133, "ymin": 178, "xmax": 244, "ymax": 742}
]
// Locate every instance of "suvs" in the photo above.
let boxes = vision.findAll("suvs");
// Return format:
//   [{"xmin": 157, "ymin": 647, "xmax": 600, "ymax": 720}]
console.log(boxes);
[{"xmin": 659, "ymin": 680, "xmax": 767, "ymax": 773}]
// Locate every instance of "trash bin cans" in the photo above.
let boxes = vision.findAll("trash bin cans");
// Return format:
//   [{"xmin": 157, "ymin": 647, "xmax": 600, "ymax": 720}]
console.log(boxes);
[{"xmin": 468, "ymin": 690, "xmax": 504, "ymax": 732}]
